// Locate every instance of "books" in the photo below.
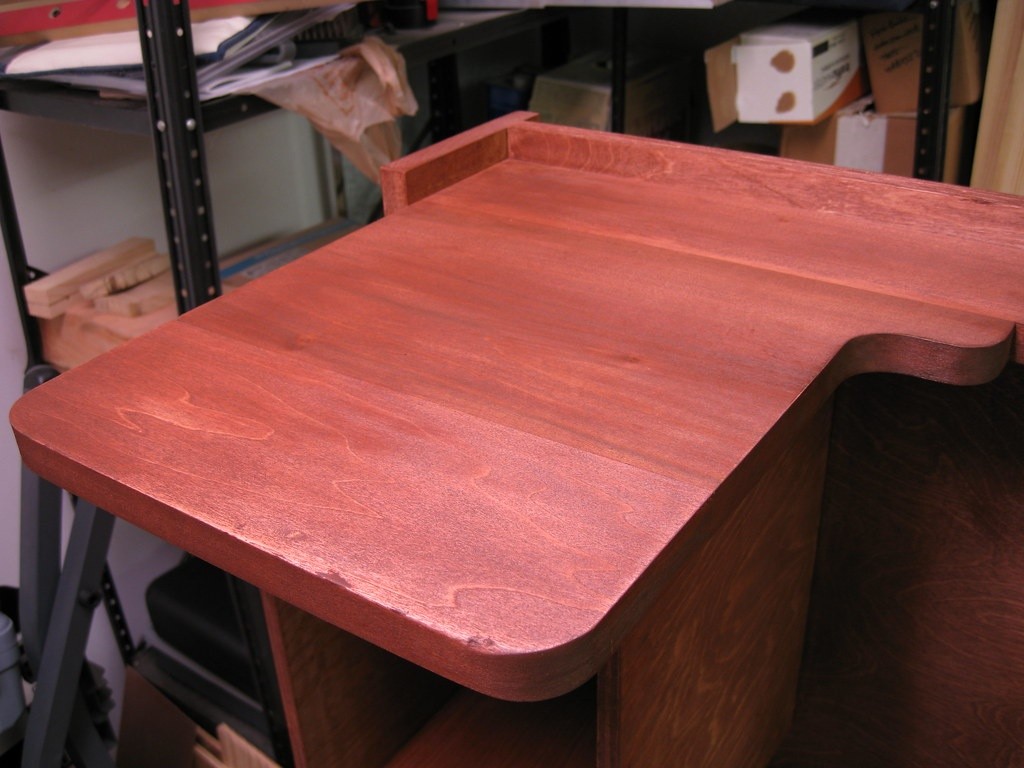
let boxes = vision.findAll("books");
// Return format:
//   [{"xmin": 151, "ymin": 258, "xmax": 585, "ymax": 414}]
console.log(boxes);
[{"xmin": 6, "ymin": 3, "xmax": 356, "ymax": 93}]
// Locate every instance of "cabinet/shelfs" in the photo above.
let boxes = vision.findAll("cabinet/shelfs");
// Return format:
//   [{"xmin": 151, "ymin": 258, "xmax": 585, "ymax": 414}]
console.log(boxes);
[{"xmin": 0, "ymin": 0, "xmax": 955, "ymax": 768}]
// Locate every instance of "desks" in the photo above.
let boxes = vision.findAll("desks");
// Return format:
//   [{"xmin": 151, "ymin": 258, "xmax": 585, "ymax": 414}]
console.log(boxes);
[{"xmin": 5, "ymin": 105, "xmax": 1024, "ymax": 768}]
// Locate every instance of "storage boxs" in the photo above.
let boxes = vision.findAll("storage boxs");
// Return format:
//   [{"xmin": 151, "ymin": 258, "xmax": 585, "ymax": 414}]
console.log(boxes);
[
  {"xmin": 703, "ymin": 13, "xmax": 871, "ymax": 129},
  {"xmin": 531, "ymin": 43, "xmax": 691, "ymax": 140},
  {"xmin": 858, "ymin": 0, "xmax": 994, "ymax": 115},
  {"xmin": 776, "ymin": 90, "xmax": 981, "ymax": 188}
]
